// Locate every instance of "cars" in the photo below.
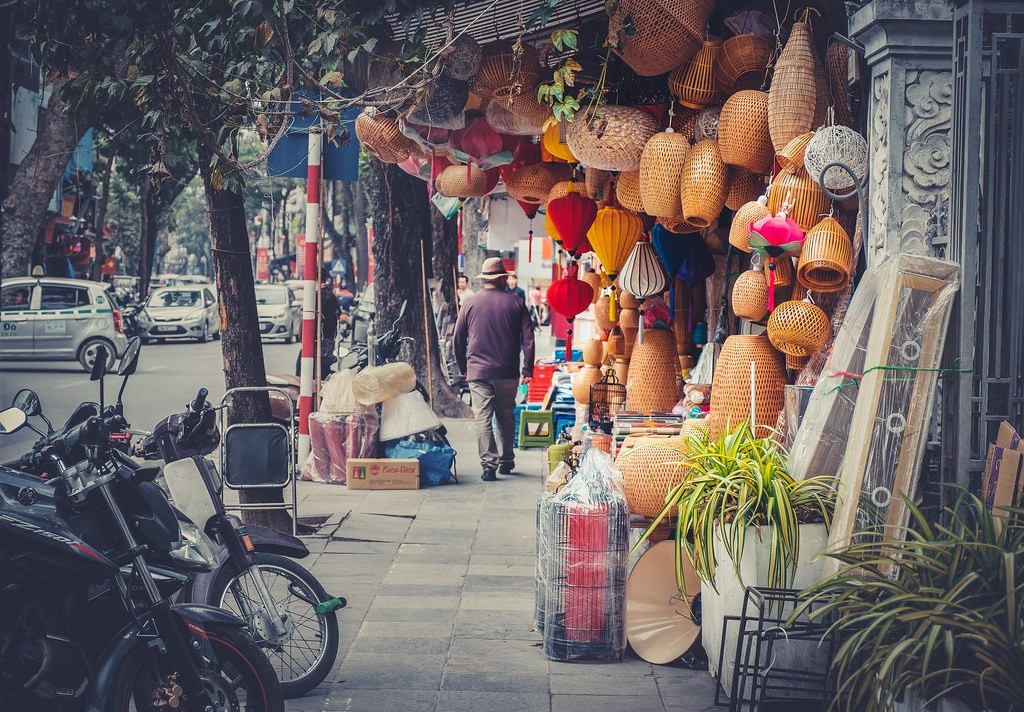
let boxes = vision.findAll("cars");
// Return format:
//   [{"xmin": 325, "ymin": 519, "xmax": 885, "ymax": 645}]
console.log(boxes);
[
  {"xmin": 109, "ymin": 273, "xmax": 213, "ymax": 312},
  {"xmin": 138, "ymin": 284, "xmax": 221, "ymax": 343},
  {"xmin": 254, "ymin": 280, "xmax": 304, "ymax": 345},
  {"xmin": 0, "ymin": 275, "xmax": 130, "ymax": 376}
]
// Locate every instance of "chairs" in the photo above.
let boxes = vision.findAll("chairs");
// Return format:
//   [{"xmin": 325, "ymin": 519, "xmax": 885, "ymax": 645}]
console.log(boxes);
[{"xmin": 218, "ymin": 387, "xmax": 297, "ymax": 536}]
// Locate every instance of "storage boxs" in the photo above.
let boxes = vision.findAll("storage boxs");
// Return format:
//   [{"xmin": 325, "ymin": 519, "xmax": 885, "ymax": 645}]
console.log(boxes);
[{"xmin": 346, "ymin": 458, "xmax": 418, "ymax": 489}]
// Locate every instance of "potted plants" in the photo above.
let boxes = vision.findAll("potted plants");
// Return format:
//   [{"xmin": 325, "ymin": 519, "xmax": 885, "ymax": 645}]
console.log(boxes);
[{"xmin": 621, "ymin": 416, "xmax": 843, "ymax": 700}]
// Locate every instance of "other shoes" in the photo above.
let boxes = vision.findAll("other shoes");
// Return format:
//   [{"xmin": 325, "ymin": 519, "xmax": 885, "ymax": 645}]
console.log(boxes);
[
  {"xmin": 481, "ymin": 469, "xmax": 496, "ymax": 481},
  {"xmin": 497, "ymin": 466, "xmax": 510, "ymax": 474}
]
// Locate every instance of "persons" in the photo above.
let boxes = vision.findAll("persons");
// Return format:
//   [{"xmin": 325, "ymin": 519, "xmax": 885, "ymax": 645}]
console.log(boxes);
[
  {"xmin": 15, "ymin": 289, "xmax": 29, "ymax": 305},
  {"xmin": 296, "ymin": 266, "xmax": 337, "ymax": 381},
  {"xmin": 505, "ymin": 270, "xmax": 526, "ymax": 304},
  {"xmin": 456, "ymin": 275, "xmax": 473, "ymax": 306},
  {"xmin": 102, "ymin": 273, "xmax": 115, "ymax": 293},
  {"xmin": 530, "ymin": 284, "xmax": 548, "ymax": 331},
  {"xmin": 452, "ymin": 257, "xmax": 535, "ymax": 482}
]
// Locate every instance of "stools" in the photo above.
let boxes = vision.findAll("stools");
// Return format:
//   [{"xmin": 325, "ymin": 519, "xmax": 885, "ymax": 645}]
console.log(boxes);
[{"xmin": 493, "ymin": 364, "xmax": 575, "ymax": 450}]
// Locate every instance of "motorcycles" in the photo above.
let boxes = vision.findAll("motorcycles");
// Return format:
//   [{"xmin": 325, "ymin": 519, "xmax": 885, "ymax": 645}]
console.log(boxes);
[
  {"xmin": 303, "ymin": 298, "xmax": 431, "ymax": 405},
  {"xmin": 0, "ymin": 333, "xmax": 341, "ymax": 712}
]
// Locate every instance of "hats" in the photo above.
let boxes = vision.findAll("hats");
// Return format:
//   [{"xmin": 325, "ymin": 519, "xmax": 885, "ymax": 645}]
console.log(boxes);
[{"xmin": 474, "ymin": 256, "xmax": 516, "ymax": 280}]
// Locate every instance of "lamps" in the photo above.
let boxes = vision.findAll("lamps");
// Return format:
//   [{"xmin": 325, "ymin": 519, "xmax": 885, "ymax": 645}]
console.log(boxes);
[{"xmin": 380, "ymin": 391, "xmax": 442, "ymax": 442}]
